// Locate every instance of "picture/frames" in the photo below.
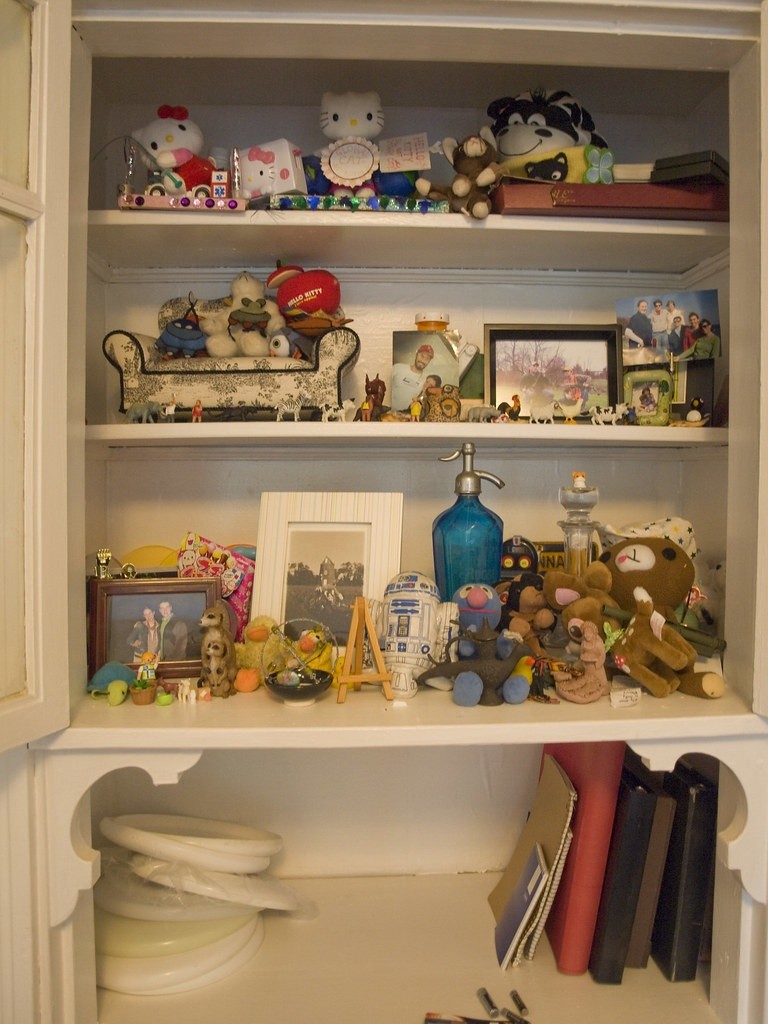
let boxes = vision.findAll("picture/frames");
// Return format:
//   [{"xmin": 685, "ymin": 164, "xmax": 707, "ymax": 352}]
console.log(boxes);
[
  {"xmin": 249, "ymin": 491, "xmax": 404, "ymax": 662},
  {"xmin": 627, "ymin": 358, "xmax": 715, "ymax": 428},
  {"xmin": 87, "ymin": 576, "xmax": 223, "ymax": 680},
  {"xmin": 623, "ymin": 370, "xmax": 672, "ymax": 427},
  {"xmin": 484, "ymin": 323, "xmax": 624, "ymax": 425}
]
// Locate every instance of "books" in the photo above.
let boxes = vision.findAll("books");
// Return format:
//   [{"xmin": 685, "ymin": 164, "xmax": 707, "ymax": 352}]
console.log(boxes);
[{"xmin": 486, "ymin": 740, "xmax": 717, "ymax": 984}]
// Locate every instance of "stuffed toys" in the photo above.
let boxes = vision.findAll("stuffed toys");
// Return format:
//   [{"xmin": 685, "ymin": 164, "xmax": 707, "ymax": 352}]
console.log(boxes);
[
  {"xmin": 132, "ymin": 105, "xmax": 216, "ymax": 191},
  {"xmin": 155, "ymin": 260, "xmax": 353, "ymax": 360},
  {"xmin": 416, "ymin": 516, "xmax": 727, "ymax": 709},
  {"xmin": 319, "ymin": 89, "xmax": 613, "ymax": 218},
  {"xmin": 196, "ymin": 599, "xmax": 355, "ymax": 699}
]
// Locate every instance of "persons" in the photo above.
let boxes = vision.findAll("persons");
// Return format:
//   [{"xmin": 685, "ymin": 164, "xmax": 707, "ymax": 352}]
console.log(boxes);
[
  {"xmin": 361, "ymin": 395, "xmax": 373, "ymax": 421},
  {"xmin": 166, "ymin": 400, "xmax": 177, "ymax": 422},
  {"xmin": 126, "ymin": 602, "xmax": 189, "ymax": 663},
  {"xmin": 520, "ymin": 362, "xmax": 592, "ymax": 413},
  {"xmin": 410, "ymin": 396, "xmax": 422, "ymax": 422},
  {"xmin": 392, "ymin": 345, "xmax": 441, "ymax": 414},
  {"xmin": 137, "ymin": 652, "xmax": 160, "ymax": 681},
  {"xmin": 192, "ymin": 400, "xmax": 202, "ymax": 423},
  {"xmin": 625, "ymin": 299, "xmax": 721, "ymax": 412}
]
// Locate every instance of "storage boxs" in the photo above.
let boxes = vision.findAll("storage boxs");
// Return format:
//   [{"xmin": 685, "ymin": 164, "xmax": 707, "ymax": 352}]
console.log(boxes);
[
  {"xmin": 229, "ymin": 138, "xmax": 308, "ymax": 207},
  {"xmin": 650, "ymin": 149, "xmax": 729, "ymax": 185},
  {"xmin": 492, "ymin": 183, "xmax": 729, "ymax": 222}
]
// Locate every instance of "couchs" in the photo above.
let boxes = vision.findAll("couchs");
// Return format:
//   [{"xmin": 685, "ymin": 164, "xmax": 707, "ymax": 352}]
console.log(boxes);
[{"xmin": 101, "ymin": 296, "xmax": 361, "ymax": 421}]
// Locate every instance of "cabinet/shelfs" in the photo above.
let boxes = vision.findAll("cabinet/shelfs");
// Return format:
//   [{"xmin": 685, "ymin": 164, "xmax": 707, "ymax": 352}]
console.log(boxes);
[{"xmin": 0, "ymin": 0, "xmax": 768, "ymax": 1024}]
